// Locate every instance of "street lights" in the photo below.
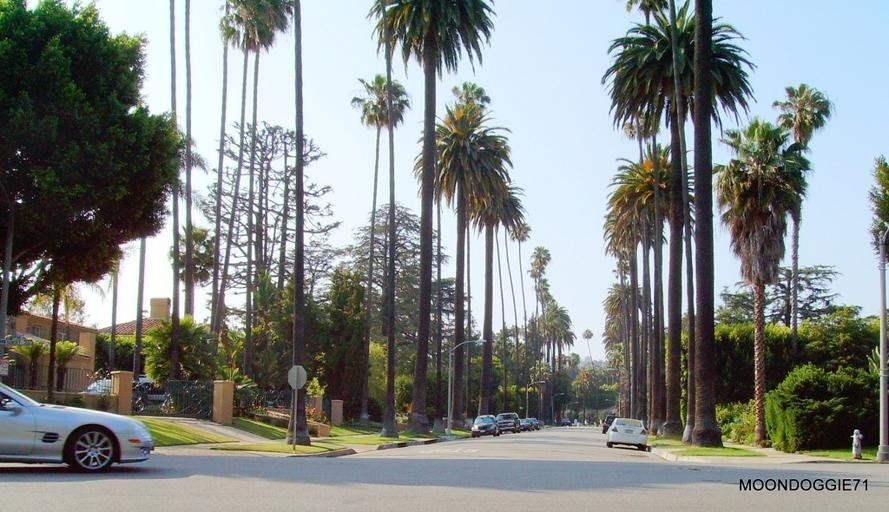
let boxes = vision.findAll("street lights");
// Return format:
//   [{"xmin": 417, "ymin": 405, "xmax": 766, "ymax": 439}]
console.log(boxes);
[
  {"xmin": 445, "ymin": 339, "xmax": 489, "ymax": 436},
  {"xmin": 525, "ymin": 380, "xmax": 546, "ymax": 419},
  {"xmin": 550, "ymin": 391, "xmax": 581, "ymax": 426}
]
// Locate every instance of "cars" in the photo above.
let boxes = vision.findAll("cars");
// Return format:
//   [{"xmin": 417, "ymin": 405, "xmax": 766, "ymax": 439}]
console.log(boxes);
[
  {"xmin": 605, "ymin": 416, "xmax": 649, "ymax": 451},
  {"xmin": 470, "ymin": 412, "xmax": 545, "ymax": 438},
  {"xmin": 0, "ymin": 382, "xmax": 156, "ymax": 473},
  {"xmin": 83, "ymin": 372, "xmax": 168, "ymax": 405}
]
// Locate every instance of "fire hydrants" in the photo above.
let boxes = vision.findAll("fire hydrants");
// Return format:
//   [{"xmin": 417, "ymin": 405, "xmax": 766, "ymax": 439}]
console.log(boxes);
[{"xmin": 850, "ymin": 428, "xmax": 865, "ymax": 459}]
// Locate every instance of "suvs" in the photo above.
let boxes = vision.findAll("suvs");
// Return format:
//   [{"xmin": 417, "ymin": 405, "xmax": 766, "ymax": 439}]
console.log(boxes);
[{"xmin": 601, "ymin": 414, "xmax": 621, "ymax": 434}]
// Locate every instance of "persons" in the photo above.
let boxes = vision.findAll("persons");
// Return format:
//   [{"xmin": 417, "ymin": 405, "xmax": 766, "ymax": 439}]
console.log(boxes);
[{"xmin": 554, "ymin": 412, "xmax": 561, "ymax": 425}]
